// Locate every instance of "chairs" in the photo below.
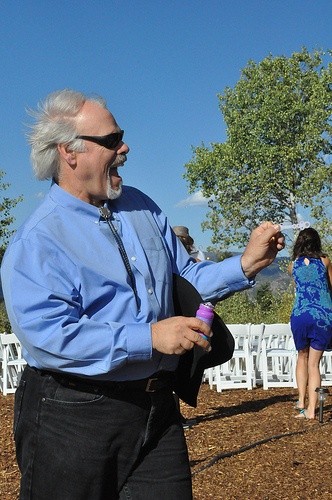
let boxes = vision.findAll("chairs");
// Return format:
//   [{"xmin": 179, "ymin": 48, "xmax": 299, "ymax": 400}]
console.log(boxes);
[
  {"xmin": 0, "ymin": 332, "xmax": 27, "ymax": 397},
  {"xmin": 201, "ymin": 322, "xmax": 332, "ymax": 393}
]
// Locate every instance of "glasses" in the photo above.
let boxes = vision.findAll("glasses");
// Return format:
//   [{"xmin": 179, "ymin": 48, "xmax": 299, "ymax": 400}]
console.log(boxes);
[{"xmin": 76, "ymin": 129, "xmax": 124, "ymax": 149}]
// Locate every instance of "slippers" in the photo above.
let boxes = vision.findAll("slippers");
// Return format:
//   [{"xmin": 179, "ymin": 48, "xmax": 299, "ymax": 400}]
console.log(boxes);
[
  {"xmin": 295, "ymin": 409, "xmax": 314, "ymax": 419},
  {"xmin": 293, "ymin": 401, "xmax": 305, "ymax": 410}
]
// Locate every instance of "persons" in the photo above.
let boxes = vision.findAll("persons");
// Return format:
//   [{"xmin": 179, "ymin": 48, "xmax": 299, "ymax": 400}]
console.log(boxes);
[
  {"xmin": 0, "ymin": 89, "xmax": 285, "ymax": 500},
  {"xmin": 173, "ymin": 225, "xmax": 205, "ymax": 263},
  {"xmin": 288, "ymin": 227, "xmax": 332, "ymax": 421}
]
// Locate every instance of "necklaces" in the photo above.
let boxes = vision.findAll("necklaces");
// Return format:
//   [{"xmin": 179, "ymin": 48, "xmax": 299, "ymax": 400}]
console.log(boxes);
[{"xmin": 99, "ymin": 204, "xmax": 140, "ymax": 309}]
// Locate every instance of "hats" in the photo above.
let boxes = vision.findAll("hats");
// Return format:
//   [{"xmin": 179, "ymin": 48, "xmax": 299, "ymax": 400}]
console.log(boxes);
[
  {"xmin": 173, "ymin": 226, "xmax": 195, "ymax": 246},
  {"xmin": 171, "ymin": 274, "xmax": 233, "ymax": 409}
]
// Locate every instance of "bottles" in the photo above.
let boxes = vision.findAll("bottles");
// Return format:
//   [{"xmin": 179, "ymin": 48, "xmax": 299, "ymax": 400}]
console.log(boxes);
[{"xmin": 195, "ymin": 302, "xmax": 215, "ymax": 343}]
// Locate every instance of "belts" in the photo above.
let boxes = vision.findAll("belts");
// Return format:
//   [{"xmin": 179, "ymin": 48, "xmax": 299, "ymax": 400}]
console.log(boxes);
[{"xmin": 26, "ymin": 363, "xmax": 178, "ymax": 393}]
